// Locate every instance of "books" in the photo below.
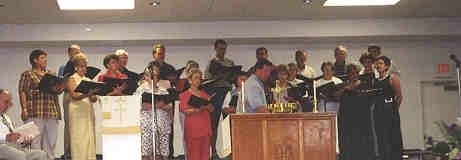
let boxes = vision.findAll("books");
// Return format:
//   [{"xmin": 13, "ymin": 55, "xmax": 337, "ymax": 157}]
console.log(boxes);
[
  {"xmin": 39, "ymin": 72, "xmax": 72, "ymax": 95},
  {"xmin": 228, "ymin": 94, "xmax": 239, "ymax": 107},
  {"xmin": 98, "ymin": 75, "xmax": 137, "ymax": 96},
  {"xmin": 209, "ymin": 59, "xmax": 242, "ymax": 77},
  {"xmin": 190, "ymin": 94, "xmax": 216, "ymax": 108},
  {"xmin": 316, "ymin": 81, "xmax": 337, "ymax": 101},
  {"xmin": 160, "ymin": 67, "xmax": 186, "ymax": 81},
  {"xmin": 119, "ymin": 65, "xmax": 144, "ymax": 83},
  {"xmin": 287, "ymin": 81, "xmax": 305, "ymax": 97},
  {"xmin": 74, "ymin": 79, "xmax": 104, "ymax": 94},
  {"xmin": 141, "ymin": 90, "xmax": 170, "ymax": 105},
  {"xmin": 14, "ymin": 121, "xmax": 40, "ymax": 143},
  {"xmin": 63, "ymin": 60, "xmax": 101, "ymax": 80}
]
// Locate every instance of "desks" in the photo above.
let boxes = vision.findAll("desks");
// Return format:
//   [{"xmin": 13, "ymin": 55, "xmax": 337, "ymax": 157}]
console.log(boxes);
[{"xmin": 230, "ymin": 111, "xmax": 336, "ymax": 160}]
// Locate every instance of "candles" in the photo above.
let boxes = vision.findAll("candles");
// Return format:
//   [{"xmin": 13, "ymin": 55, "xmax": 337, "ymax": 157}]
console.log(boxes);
[
  {"xmin": 241, "ymin": 81, "xmax": 244, "ymax": 104},
  {"xmin": 314, "ymin": 80, "xmax": 316, "ymax": 100}
]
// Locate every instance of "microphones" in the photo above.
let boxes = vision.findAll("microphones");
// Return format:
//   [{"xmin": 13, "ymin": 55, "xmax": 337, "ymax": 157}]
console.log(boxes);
[{"xmin": 448, "ymin": 53, "xmax": 461, "ymax": 65}]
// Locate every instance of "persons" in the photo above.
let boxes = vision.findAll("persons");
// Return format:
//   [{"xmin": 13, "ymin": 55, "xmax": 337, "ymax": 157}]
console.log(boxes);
[
  {"xmin": 18, "ymin": 50, "xmax": 61, "ymax": 160},
  {"xmin": 0, "ymin": 89, "xmax": 51, "ymax": 160},
  {"xmin": 311, "ymin": 43, "xmax": 403, "ymax": 160},
  {"xmin": 204, "ymin": 39, "xmax": 315, "ymax": 160},
  {"xmin": 58, "ymin": 44, "xmax": 97, "ymax": 160},
  {"xmin": 98, "ymin": 48, "xmax": 128, "ymax": 104},
  {"xmin": 137, "ymin": 43, "xmax": 214, "ymax": 160}
]
